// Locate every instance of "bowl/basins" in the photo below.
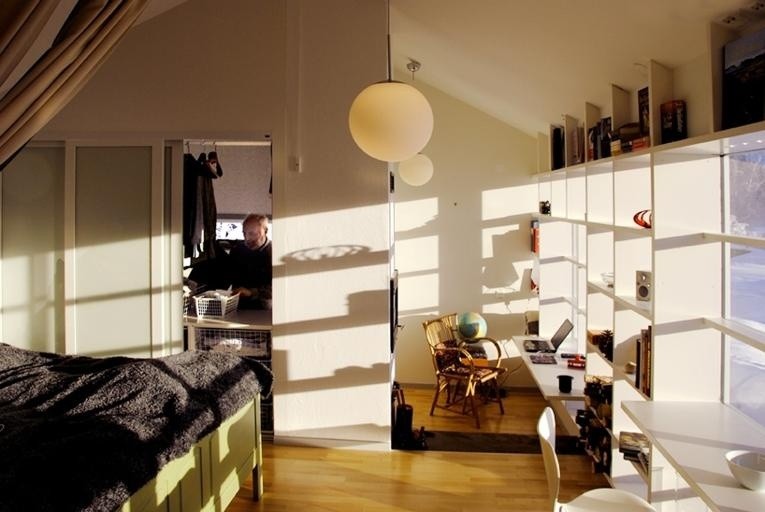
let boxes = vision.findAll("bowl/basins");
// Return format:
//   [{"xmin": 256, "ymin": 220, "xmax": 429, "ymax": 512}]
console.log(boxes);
[{"xmin": 725, "ymin": 447, "xmax": 765, "ymax": 492}]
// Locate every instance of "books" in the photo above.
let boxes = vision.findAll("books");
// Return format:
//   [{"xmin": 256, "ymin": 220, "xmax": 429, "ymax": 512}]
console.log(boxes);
[
  {"xmin": 619, "ymin": 430, "xmax": 650, "ymax": 479},
  {"xmin": 551, "ymin": 97, "xmax": 689, "ymax": 171},
  {"xmin": 635, "ymin": 325, "xmax": 653, "ymax": 401},
  {"xmin": 530, "ymin": 220, "xmax": 539, "ymax": 253}
]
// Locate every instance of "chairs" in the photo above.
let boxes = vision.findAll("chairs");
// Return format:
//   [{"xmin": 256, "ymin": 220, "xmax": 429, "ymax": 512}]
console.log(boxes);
[{"xmin": 422, "ymin": 312, "xmax": 506, "ymax": 429}]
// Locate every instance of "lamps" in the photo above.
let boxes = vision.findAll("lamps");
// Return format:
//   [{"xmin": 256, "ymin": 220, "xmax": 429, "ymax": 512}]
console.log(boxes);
[{"xmin": 347, "ymin": 0, "xmax": 435, "ymax": 188}]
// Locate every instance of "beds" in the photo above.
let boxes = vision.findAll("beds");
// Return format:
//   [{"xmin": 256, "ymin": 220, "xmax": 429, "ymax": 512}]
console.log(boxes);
[{"xmin": 0, "ymin": 343, "xmax": 274, "ymax": 512}]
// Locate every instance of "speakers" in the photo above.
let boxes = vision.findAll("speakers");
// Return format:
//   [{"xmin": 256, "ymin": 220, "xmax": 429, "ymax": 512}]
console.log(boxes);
[{"xmin": 636, "ymin": 271, "xmax": 651, "ymax": 301}]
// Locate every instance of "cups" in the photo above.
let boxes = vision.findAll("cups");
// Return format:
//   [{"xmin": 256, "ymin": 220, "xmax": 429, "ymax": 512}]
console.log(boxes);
[{"xmin": 558, "ymin": 374, "xmax": 574, "ymax": 393}]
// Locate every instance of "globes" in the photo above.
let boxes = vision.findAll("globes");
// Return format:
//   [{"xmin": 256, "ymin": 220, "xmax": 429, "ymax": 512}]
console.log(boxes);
[{"xmin": 458, "ymin": 313, "xmax": 487, "ymax": 343}]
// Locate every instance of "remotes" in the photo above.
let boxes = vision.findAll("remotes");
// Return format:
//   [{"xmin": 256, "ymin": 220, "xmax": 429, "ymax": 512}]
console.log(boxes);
[{"xmin": 561, "ymin": 353, "xmax": 585, "ymax": 359}]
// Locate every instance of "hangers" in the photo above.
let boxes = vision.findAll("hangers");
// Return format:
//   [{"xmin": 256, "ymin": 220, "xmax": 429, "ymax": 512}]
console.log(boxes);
[{"xmin": 184, "ymin": 135, "xmax": 225, "ymax": 179}]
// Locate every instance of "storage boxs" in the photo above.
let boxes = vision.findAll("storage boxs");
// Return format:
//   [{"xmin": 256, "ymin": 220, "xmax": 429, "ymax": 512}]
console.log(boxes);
[{"xmin": 183, "ymin": 279, "xmax": 270, "ymax": 358}]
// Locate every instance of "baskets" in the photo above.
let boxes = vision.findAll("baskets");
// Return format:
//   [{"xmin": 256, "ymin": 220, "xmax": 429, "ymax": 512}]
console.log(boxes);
[{"xmin": 194, "ymin": 289, "xmax": 241, "ymax": 319}]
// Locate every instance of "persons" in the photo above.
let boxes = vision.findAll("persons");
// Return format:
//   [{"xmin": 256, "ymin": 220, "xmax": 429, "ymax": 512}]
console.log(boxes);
[
  {"xmin": 187, "ymin": 240, "xmax": 231, "ymax": 294},
  {"xmin": 228, "ymin": 213, "xmax": 272, "ymax": 311}
]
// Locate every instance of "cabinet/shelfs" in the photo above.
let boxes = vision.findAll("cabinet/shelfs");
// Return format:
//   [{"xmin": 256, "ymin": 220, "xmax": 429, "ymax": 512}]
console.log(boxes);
[{"xmin": 512, "ymin": 32, "xmax": 765, "ymax": 512}]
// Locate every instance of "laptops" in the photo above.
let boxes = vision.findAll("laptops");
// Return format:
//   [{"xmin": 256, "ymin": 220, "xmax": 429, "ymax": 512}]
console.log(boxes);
[{"xmin": 523, "ymin": 318, "xmax": 574, "ymax": 352}]
